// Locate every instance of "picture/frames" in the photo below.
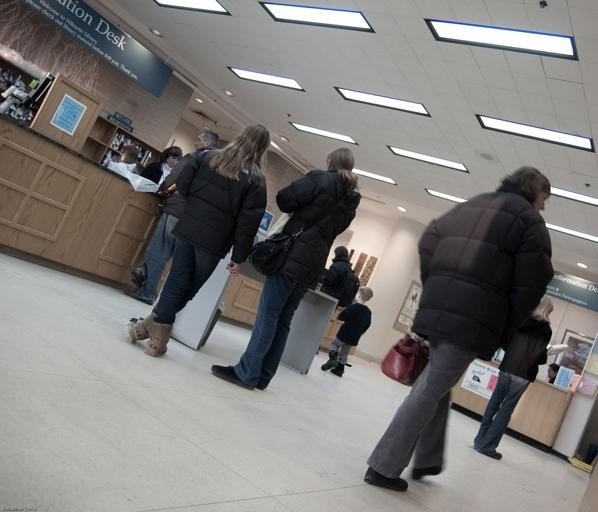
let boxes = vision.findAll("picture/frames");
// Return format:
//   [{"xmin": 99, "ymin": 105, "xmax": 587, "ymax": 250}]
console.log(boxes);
[
  {"xmin": 549, "ymin": 328, "xmax": 596, "ymax": 377},
  {"xmin": 257, "ymin": 208, "xmax": 275, "ymax": 235}
]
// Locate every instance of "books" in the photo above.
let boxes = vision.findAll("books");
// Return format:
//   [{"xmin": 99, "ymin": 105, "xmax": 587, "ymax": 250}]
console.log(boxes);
[{"xmin": 107, "ymin": 161, "xmax": 159, "ymax": 193}]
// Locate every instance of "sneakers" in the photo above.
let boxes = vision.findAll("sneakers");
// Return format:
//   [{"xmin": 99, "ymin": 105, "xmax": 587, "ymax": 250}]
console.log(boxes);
[
  {"xmin": 132, "ymin": 269, "xmax": 144, "ymax": 287},
  {"xmin": 413, "ymin": 466, "xmax": 442, "ymax": 479},
  {"xmin": 487, "ymin": 448, "xmax": 503, "ymax": 459},
  {"xmin": 212, "ymin": 365, "xmax": 264, "ymax": 390},
  {"xmin": 366, "ymin": 468, "xmax": 408, "ymax": 492}
]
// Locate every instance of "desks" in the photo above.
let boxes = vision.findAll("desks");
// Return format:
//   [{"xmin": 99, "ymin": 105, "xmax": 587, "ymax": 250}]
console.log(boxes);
[{"xmin": 145, "ymin": 243, "xmax": 341, "ymax": 376}]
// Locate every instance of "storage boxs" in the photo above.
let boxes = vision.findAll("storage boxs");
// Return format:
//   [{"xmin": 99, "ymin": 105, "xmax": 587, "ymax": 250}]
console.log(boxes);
[{"xmin": 29, "ymin": 71, "xmax": 106, "ymax": 157}]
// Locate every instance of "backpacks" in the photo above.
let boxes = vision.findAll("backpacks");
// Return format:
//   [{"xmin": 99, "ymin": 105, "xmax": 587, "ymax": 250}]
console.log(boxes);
[{"xmin": 338, "ymin": 262, "xmax": 360, "ymax": 307}]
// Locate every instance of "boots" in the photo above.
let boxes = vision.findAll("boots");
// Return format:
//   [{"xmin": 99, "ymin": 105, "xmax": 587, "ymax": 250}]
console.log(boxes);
[
  {"xmin": 331, "ymin": 363, "xmax": 344, "ymax": 377},
  {"xmin": 145, "ymin": 322, "xmax": 172, "ymax": 358},
  {"xmin": 322, "ymin": 351, "xmax": 337, "ymax": 370},
  {"xmin": 128, "ymin": 312, "xmax": 156, "ymax": 343}
]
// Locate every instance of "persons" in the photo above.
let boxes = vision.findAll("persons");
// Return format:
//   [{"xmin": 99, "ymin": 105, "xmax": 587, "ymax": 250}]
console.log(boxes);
[
  {"xmin": 321, "ymin": 286, "xmax": 373, "ymax": 378},
  {"xmin": 473, "ymin": 295, "xmax": 553, "ymax": 460},
  {"xmin": 124, "ymin": 130, "xmax": 220, "ymax": 305},
  {"xmin": 128, "ymin": 122, "xmax": 271, "ymax": 358},
  {"xmin": 363, "ymin": 165, "xmax": 553, "ymax": 491},
  {"xmin": 320, "ymin": 246, "xmax": 351, "ymax": 298},
  {"xmin": 102, "ymin": 144, "xmax": 139, "ymax": 176},
  {"xmin": 546, "ymin": 364, "xmax": 559, "ymax": 384},
  {"xmin": 140, "ymin": 146, "xmax": 182, "ymax": 186},
  {"xmin": 209, "ymin": 147, "xmax": 361, "ymax": 392}
]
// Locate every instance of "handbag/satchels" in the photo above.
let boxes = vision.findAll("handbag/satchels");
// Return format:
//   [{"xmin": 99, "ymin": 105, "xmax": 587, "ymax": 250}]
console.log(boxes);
[
  {"xmin": 249, "ymin": 232, "xmax": 292, "ymax": 276},
  {"xmin": 382, "ymin": 333, "xmax": 430, "ymax": 385}
]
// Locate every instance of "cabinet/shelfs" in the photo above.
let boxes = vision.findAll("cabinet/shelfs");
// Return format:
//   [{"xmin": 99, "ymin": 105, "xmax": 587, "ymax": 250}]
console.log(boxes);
[
  {"xmin": 318, "ymin": 304, "xmax": 357, "ymax": 358},
  {"xmin": 217, "ymin": 254, "xmax": 270, "ymax": 331},
  {"xmin": 1, "ymin": 110, "xmax": 175, "ymax": 307},
  {"xmin": 148, "ymin": 241, "xmax": 236, "ymax": 353},
  {"xmin": 446, "ymin": 353, "xmax": 573, "ymax": 449}
]
